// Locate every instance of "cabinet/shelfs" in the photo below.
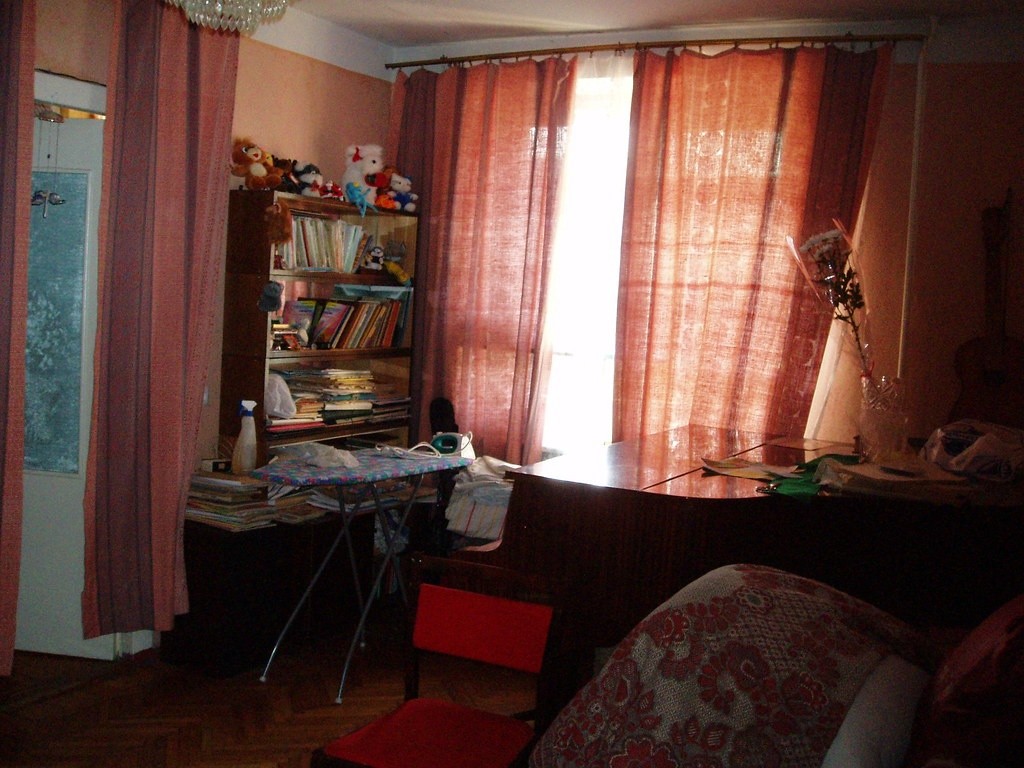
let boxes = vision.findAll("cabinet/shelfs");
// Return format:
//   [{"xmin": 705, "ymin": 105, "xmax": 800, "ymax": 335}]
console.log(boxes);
[
  {"xmin": 218, "ymin": 189, "xmax": 423, "ymax": 471},
  {"xmin": 257, "ymin": 447, "xmax": 470, "ymax": 704}
]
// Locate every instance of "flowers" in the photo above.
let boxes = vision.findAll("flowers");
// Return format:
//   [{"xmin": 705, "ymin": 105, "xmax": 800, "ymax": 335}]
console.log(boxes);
[{"xmin": 793, "ymin": 220, "xmax": 899, "ymax": 410}]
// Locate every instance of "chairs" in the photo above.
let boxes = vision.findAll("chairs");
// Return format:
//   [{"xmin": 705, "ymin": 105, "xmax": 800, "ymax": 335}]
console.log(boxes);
[
  {"xmin": 311, "ymin": 553, "xmax": 571, "ymax": 768},
  {"xmin": 410, "ymin": 474, "xmax": 528, "ymax": 707}
]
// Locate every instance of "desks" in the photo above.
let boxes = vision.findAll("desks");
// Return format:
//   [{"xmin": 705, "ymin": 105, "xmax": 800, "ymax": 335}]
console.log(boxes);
[
  {"xmin": 162, "ymin": 468, "xmax": 453, "ymax": 672},
  {"xmin": 507, "ymin": 424, "xmax": 858, "ymax": 698}
]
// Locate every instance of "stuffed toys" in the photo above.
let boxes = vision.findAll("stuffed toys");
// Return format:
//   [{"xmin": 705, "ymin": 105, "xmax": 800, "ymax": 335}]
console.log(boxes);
[
  {"xmin": 256, "ymin": 280, "xmax": 283, "ymax": 311},
  {"xmin": 232, "ymin": 137, "xmax": 419, "ymax": 216},
  {"xmin": 367, "ymin": 247, "xmax": 384, "ymax": 271},
  {"xmin": 265, "ymin": 200, "xmax": 292, "ymax": 243}
]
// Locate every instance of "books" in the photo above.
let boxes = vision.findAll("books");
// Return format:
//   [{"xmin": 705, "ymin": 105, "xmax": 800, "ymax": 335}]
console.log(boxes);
[{"xmin": 183, "ymin": 215, "xmax": 441, "ymax": 526}]
